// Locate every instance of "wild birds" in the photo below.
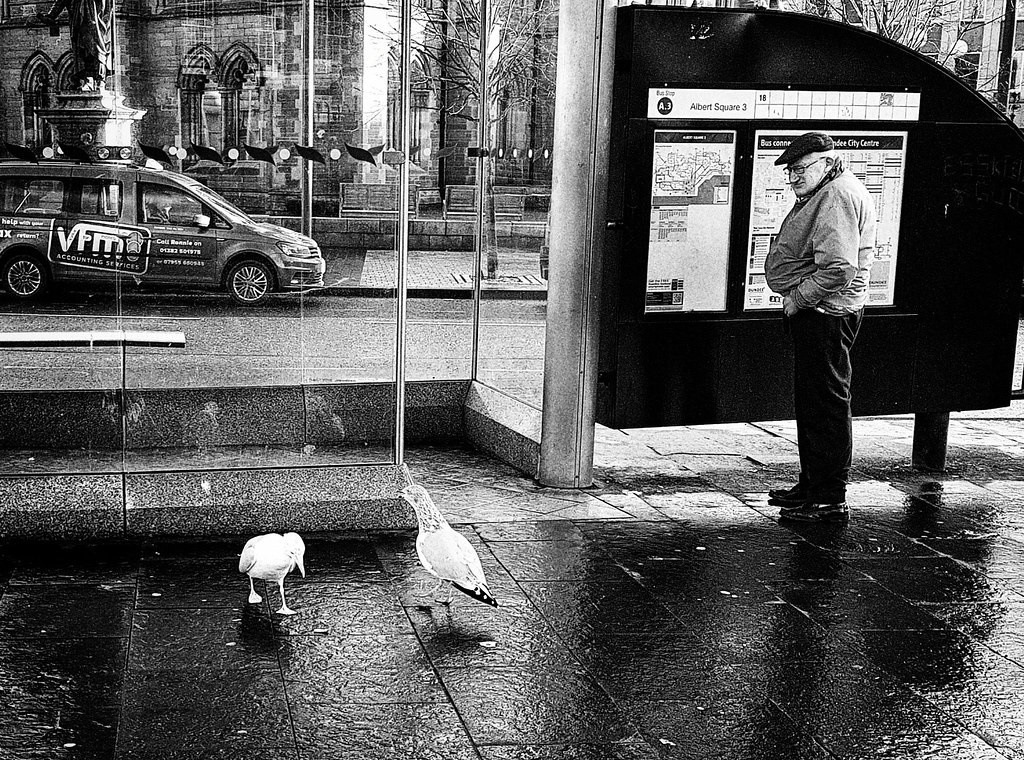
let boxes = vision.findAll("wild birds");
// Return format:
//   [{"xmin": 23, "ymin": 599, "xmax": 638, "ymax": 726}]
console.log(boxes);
[
  {"xmin": 238, "ymin": 532, "xmax": 306, "ymax": 615},
  {"xmin": 397, "ymin": 483, "xmax": 499, "ymax": 610}
]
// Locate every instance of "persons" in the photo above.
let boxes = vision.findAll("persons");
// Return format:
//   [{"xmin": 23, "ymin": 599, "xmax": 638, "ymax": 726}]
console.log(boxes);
[
  {"xmin": 762, "ymin": 133, "xmax": 876, "ymax": 525},
  {"xmin": 146, "ymin": 193, "xmax": 173, "ymax": 225}
]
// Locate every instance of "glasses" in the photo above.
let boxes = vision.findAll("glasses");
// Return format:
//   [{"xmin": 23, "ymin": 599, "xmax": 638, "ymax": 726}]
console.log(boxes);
[{"xmin": 783, "ymin": 157, "xmax": 827, "ymax": 174}]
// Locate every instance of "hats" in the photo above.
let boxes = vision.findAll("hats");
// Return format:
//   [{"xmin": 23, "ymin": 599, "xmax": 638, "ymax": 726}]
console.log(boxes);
[{"xmin": 773, "ymin": 132, "xmax": 834, "ymax": 166}]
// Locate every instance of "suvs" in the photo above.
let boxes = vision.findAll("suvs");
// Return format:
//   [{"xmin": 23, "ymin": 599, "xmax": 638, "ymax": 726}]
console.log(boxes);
[{"xmin": 0, "ymin": 155, "xmax": 327, "ymax": 310}]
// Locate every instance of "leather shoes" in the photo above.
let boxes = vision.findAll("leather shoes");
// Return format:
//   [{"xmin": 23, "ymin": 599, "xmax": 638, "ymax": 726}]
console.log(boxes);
[
  {"xmin": 779, "ymin": 501, "xmax": 851, "ymax": 521},
  {"xmin": 769, "ymin": 484, "xmax": 801, "ymax": 504}
]
